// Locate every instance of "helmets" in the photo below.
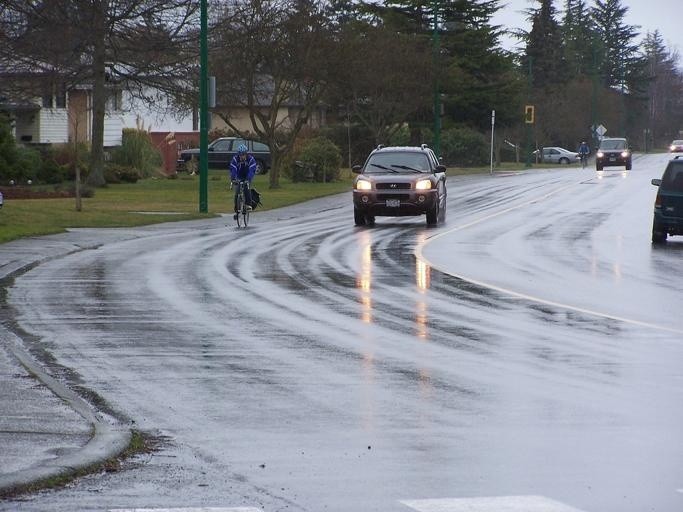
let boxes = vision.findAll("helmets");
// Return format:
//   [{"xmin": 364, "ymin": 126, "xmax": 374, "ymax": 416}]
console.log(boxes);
[{"xmin": 237, "ymin": 144, "xmax": 248, "ymax": 153}]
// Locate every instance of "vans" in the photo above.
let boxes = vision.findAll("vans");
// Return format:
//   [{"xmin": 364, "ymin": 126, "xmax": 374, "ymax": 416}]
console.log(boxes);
[{"xmin": 174, "ymin": 136, "xmax": 273, "ymax": 173}]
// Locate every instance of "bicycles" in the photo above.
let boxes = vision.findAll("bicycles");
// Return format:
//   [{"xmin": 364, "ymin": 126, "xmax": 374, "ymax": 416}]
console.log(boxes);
[
  {"xmin": 579, "ymin": 151, "xmax": 588, "ymax": 168},
  {"xmin": 228, "ymin": 180, "xmax": 252, "ymax": 228}
]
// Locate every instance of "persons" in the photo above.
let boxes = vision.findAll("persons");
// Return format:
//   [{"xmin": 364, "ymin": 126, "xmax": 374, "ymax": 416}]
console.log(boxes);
[
  {"xmin": 578, "ymin": 141, "xmax": 590, "ymax": 167},
  {"xmin": 229, "ymin": 143, "xmax": 257, "ymax": 220}
]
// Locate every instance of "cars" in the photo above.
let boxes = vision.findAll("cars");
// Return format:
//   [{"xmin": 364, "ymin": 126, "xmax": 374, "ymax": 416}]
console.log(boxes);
[
  {"xmin": 530, "ymin": 146, "xmax": 581, "ymax": 163},
  {"xmin": 594, "ymin": 138, "xmax": 631, "ymax": 171},
  {"xmin": 649, "ymin": 156, "xmax": 683, "ymax": 243},
  {"xmin": 667, "ymin": 140, "xmax": 683, "ymax": 153}
]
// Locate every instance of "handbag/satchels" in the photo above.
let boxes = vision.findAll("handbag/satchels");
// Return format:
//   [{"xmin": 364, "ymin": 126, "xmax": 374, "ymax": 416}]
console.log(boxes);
[{"xmin": 251, "ymin": 189, "xmax": 260, "ymax": 210}]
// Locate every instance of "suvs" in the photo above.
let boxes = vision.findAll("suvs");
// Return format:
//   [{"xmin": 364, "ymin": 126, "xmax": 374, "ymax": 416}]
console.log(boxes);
[{"xmin": 347, "ymin": 144, "xmax": 449, "ymax": 227}]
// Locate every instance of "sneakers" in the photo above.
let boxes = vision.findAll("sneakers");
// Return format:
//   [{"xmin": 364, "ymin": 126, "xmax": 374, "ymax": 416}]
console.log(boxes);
[
  {"xmin": 246, "ymin": 205, "xmax": 252, "ymax": 210},
  {"xmin": 234, "ymin": 213, "xmax": 237, "ymax": 216}
]
